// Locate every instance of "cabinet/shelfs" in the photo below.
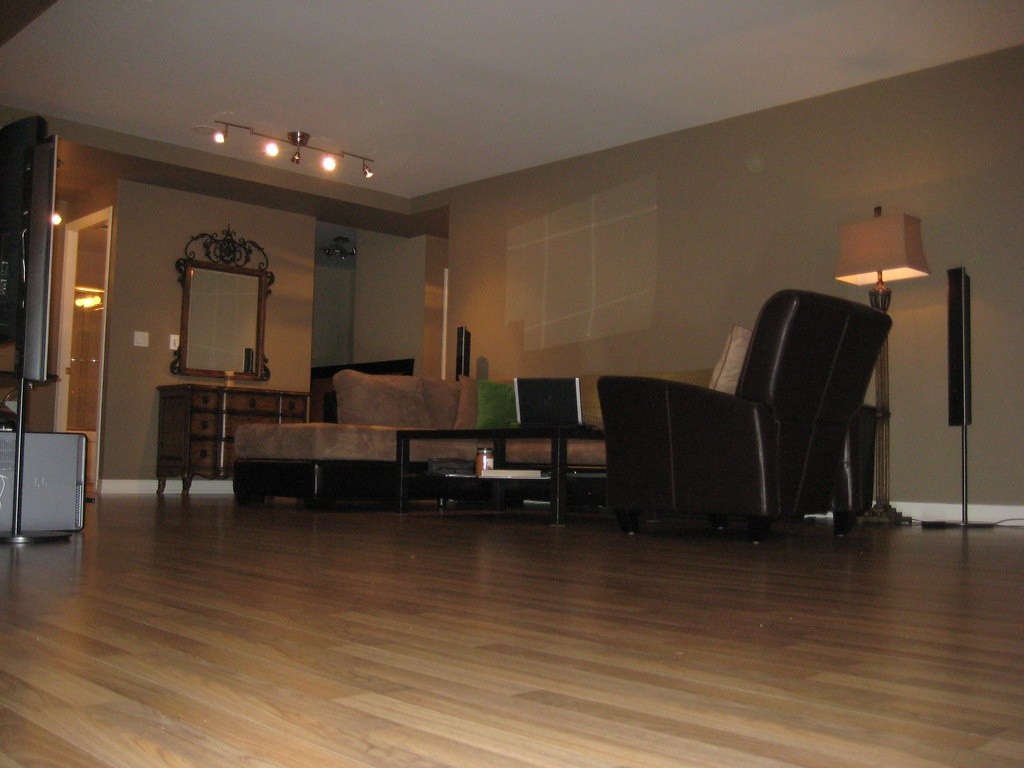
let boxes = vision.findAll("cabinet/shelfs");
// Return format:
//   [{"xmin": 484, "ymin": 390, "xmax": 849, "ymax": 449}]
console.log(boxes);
[{"xmin": 155, "ymin": 383, "xmax": 312, "ymax": 497}]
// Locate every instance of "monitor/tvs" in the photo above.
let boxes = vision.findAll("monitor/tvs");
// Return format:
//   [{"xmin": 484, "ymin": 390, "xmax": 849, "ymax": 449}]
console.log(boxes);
[{"xmin": 0, "ymin": 116, "xmax": 48, "ymax": 344}]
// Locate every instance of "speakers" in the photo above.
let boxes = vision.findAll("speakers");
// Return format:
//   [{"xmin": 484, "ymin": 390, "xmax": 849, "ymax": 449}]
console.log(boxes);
[
  {"xmin": 948, "ymin": 267, "xmax": 971, "ymax": 423},
  {"xmin": 455, "ymin": 326, "xmax": 471, "ymax": 381},
  {"xmin": 16, "ymin": 136, "xmax": 62, "ymax": 381}
]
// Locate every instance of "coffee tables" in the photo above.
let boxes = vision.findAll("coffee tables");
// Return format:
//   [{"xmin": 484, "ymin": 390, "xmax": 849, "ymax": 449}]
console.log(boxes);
[{"xmin": 396, "ymin": 424, "xmax": 603, "ymax": 528}]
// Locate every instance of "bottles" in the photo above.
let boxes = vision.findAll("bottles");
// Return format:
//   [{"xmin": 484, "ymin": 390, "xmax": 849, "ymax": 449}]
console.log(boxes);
[{"xmin": 224, "ymin": 372, "xmax": 235, "ymax": 387}]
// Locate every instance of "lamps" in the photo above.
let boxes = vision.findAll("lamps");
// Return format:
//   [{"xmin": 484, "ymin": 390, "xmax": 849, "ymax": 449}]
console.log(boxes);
[
  {"xmin": 319, "ymin": 235, "xmax": 356, "ymax": 260},
  {"xmin": 213, "ymin": 120, "xmax": 375, "ymax": 178},
  {"xmin": 834, "ymin": 204, "xmax": 931, "ymax": 527}
]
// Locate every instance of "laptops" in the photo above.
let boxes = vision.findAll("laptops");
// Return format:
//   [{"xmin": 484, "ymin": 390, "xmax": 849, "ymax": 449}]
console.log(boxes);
[{"xmin": 514, "ymin": 378, "xmax": 584, "ymax": 425}]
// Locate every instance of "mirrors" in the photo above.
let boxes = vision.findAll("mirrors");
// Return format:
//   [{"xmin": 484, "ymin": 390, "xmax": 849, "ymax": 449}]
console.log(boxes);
[{"xmin": 169, "ymin": 223, "xmax": 276, "ymax": 381}]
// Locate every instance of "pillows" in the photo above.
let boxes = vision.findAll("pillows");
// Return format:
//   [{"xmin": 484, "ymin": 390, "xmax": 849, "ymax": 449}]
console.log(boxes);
[
  {"xmin": 420, "ymin": 377, "xmax": 461, "ymax": 429},
  {"xmin": 475, "ymin": 380, "xmax": 519, "ymax": 428},
  {"xmin": 708, "ymin": 325, "xmax": 753, "ymax": 396},
  {"xmin": 453, "ymin": 374, "xmax": 477, "ymax": 430}
]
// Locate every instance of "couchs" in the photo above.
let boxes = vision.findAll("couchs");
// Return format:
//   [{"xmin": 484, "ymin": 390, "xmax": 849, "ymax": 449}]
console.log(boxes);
[
  {"xmin": 232, "ymin": 369, "xmax": 714, "ymax": 515},
  {"xmin": 598, "ymin": 288, "xmax": 893, "ymax": 536}
]
